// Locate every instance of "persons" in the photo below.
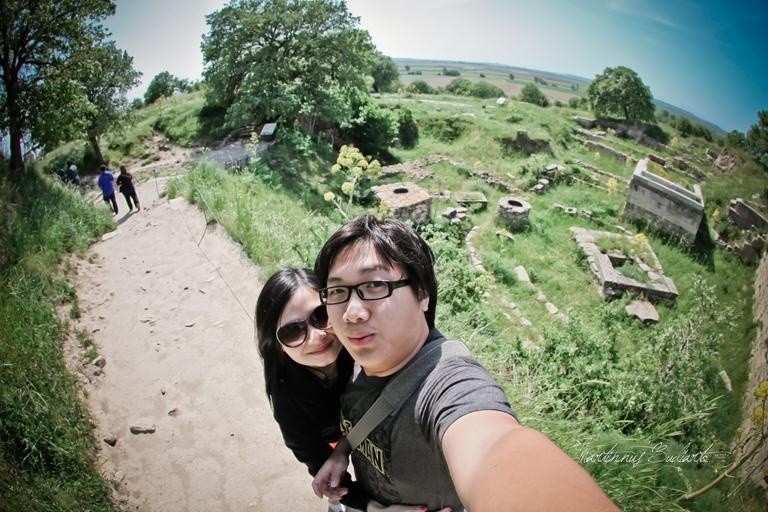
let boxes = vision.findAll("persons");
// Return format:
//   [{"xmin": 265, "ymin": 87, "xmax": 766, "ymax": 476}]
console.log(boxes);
[
  {"xmin": 115, "ymin": 166, "xmax": 141, "ymax": 211},
  {"xmin": 98, "ymin": 163, "xmax": 119, "ymax": 214},
  {"xmin": 311, "ymin": 210, "xmax": 625, "ymax": 512},
  {"xmin": 56, "ymin": 161, "xmax": 81, "ymax": 185},
  {"xmin": 252, "ymin": 266, "xmax": 453, "ymax": 512}
]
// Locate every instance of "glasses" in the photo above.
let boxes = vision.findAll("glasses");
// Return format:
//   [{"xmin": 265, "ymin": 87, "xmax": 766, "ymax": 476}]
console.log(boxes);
[
  {"xmin": 320, "ymin": 278, "xmax": 410, "ymax": 305},
  {"xmin": 276, "ymin": 303, "xmax": 332, "ymax": 349}
]
